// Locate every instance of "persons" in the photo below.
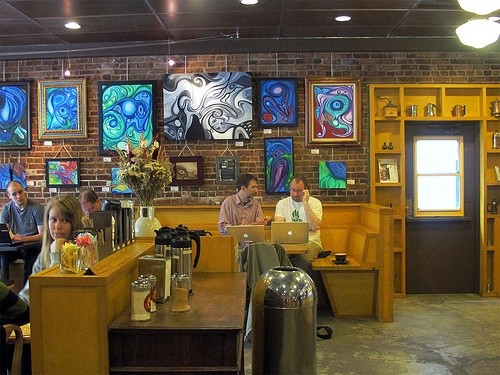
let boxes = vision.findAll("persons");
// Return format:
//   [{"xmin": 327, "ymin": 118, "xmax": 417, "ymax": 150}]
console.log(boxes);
[
  {"xmin": 0, "ymin": 281, "xmax": 34, "ymax": 330},
  {"xmin": 218, "ymin": 174, "xmax": 269, "ymax": 236},
  {"xmin": 274, "ymin": 174, "xmax": 323, "ymax": 275},
  {"xmin": 17, "ymin": 194, "xmax": 88, "ymax": 308},
  {"xmin": 77, "ymin": 188, "xmax": 111, "ymax": 218},
  {"xmin": 0, "ymin": 181, "xmax": 44, "ymax": 292}
]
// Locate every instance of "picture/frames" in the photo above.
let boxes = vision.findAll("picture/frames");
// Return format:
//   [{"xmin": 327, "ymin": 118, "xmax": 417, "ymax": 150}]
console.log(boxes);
[
  {"xmin": 305, "ymin": 76, "xmax": 364, "ymax": 148},
  {"xmin": 377, "ymin": 158, "xmax": 396, "ymax": 182},
  {"xmin": 161, "ymin": 71, "xmax": 254, "ymax": 141},
  {"xmin": 110, "ymin": 167, "xmax": 136, "ymax": 194},
  {"xmin": 379, "ymin": 163, "xmax": 399, "ymax": 184},
  {"xmin": 45, "ymin": 158, "xmax": 81, "ymax": 188},
  {"xmin": 0, "ymin": 163, "xmax": 28, "ymax": 191},
  {"xmin": 97, "ymin": 79, "xmax": 157, "ymax": 157},
  {"xmin": 37, "ymin": 77, "xmax": 88, "ymax": 141},
  {"xmin": 318, "ymin": 159, "xmax": 348, "ymax": 191},
  {"xmin": 0, "ymin": 81, "xmax": 33, "ymax": 151},
  {"xmin": 255, "ymin": 77, "xmax": 298, "ymax": 128},
  {"xmin": 263, "ymin": 136, "xmax": 294, "ymax": 194},
  {"xmin": 167, "ymin": 156, "xmax": 205, "ymax": 186},
  {"xmin": 215, "ymin": 155, "xmax": 239, "ymax": 184}
]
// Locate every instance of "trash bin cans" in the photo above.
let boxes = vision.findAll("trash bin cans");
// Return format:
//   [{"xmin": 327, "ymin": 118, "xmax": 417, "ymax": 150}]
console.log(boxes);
[{"xmin": 251, "ymin": 266, "xmax": 318, "ymax": 375}]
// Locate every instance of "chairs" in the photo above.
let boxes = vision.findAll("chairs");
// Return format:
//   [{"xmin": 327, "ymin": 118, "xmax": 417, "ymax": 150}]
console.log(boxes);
[
  {"xmin": 0, "ymin": 324, "xmax": 24, "ymax": 375},
  {"xmin": 0, "ymin": 221, "xmax": 24, "ymax": 264}
]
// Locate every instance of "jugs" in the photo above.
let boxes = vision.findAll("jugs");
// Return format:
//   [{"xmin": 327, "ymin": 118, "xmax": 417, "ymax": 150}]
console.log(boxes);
[
  {"xmin": 154, "ymin": 225, "xmax": 201, "ymax": 296},
  {"xmin": 490, "ymin": 98, "xmax": 500, "ymax": 117},
  {"xmin": 425, "ymin": 103, "xmax": 439, "ymax": 116}
]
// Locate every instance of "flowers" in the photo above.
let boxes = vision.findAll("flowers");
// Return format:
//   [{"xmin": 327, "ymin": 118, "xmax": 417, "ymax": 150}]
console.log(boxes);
[{"xmin": 111, "ymin": 132, "xmax": 172, "ymax": 205}]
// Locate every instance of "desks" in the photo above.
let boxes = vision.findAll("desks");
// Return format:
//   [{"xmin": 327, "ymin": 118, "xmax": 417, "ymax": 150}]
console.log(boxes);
[
  {"xmin": 107, "ymin": 271, "xmax": 248, "ymax": 374},
  {"xmin": 282, "ymin": 242, "xmax": 308, "ymax": 266}
]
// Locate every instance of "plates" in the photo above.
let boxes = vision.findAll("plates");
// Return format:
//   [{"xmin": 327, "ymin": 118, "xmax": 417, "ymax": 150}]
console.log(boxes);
[{"xmin": 332, "ymin": 260, "xmax": 350, "ymax": 264}]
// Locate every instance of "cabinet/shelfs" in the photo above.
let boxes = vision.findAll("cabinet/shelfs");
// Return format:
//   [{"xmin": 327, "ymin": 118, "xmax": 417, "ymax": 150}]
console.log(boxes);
[{"xmin": 368, "ymin": 82, "xmax": 499, "ymax": 299}]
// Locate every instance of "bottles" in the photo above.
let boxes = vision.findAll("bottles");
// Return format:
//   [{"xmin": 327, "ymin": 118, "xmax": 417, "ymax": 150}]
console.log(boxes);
[
  {"xmin": 130, "ymin": 280, "xmax": 151, "ymax": 321},
  {"xmin": 170, "ymin": 272, "xmax": 191, "ymax": 312},
  {"xmin": 138, "ymin": 273, "xmax": 158, "ymax": 313}
]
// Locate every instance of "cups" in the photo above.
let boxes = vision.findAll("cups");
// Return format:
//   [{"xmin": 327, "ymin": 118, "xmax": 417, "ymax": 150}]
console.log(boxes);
[
  {"xmin": 406, "ymin": 104, "xmax": 418, "ymax": 117},
  {"xmin": 452, "ymin": 105, "xmax": 466, "ymax": 117},
  {"xmin": 335, "ymin": 253, "xmax": 347, "ymax": 262}
]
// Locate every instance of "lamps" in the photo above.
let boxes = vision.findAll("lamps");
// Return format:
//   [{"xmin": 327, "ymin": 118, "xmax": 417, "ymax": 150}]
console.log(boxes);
[
  {"xmin": 454, "ymin": 13, "xmax": 500, "ymax": 50},
  {"xmin": 456, "ymin": 0, "xmax": 500, "ymax": 16}
]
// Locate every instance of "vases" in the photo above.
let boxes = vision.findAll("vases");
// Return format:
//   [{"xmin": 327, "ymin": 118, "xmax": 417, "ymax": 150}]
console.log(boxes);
[{"xmin": 133, "ymin": 206, "xmax": 163, "ymax": 239}]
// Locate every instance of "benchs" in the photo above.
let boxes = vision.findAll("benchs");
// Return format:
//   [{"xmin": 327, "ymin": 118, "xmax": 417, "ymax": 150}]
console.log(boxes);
[{"xmin": 310, "ymin": 251, "xmax": 360, "ymax": 271}]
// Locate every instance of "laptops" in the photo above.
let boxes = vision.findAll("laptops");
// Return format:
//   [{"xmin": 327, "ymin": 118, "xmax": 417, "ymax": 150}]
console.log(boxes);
[
  {"xmin": 270, "ymin": 221, "xmax": 309, "ymax": 244},
  {"xmin": 226, "ymin": 225, "xmax": 266, "ymax": 244},
  {"xmin": 0, "ymin": 223, "xmax": 24, "ymax": 246}
]
